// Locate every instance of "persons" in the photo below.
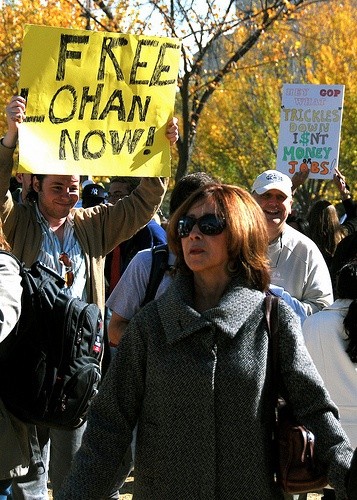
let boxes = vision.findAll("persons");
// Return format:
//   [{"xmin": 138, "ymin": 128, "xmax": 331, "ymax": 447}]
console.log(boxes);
[
  {"xmin": 303, "ymin": 262, "xmax": 357, "ymax": 450},
  {"xmin": 0, "ymin": 169, "xmax": 357, "ymax": 450},
  {"xmin": 344, "ymin": 449, "xmax": 357, "ymax": 500},
  {"xmin": 53, "ymin": 182, "xmax": 354, "ymax": 500},
  {"xmin": 0, "ymin": 217, "xmax": 33, "ymax": 500},
  {"xmin": 0, "ymin": 95, "xmax": 180, "ymax": 496}
]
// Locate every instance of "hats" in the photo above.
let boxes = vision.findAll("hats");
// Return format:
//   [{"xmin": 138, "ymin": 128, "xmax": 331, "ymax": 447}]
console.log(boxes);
[
  {"xmin": 251, "ymin": 169, "xmax": 293, "ymax": 197},
  {"xmin": 81, "ymin": 184, "xmax": 105, "ymax": 200}
]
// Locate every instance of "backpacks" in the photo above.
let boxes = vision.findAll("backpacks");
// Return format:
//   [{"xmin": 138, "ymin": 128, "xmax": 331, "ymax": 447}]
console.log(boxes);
[{"xmin": 0, "ymin": 250, "xmax": 103, "ymax": 430}]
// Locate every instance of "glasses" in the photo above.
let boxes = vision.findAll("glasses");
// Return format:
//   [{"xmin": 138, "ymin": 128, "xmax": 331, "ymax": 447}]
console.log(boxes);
[
  {"xmin": 59, "ymin": 251, "xmax": 74, "ymax": 289},
  {"xmin": 176, "ymin": 213, "xmax": 225, "ymax": 238},
  {"xmin": 105, "ymin": 191, "xmax": 130, "ymax": 200}
]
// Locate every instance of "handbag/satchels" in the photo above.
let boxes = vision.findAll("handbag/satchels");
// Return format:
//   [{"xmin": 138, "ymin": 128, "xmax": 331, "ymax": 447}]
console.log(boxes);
[{"xmin": 277, "ymin": 426, "xmax": 328, "ymax": 495}]
